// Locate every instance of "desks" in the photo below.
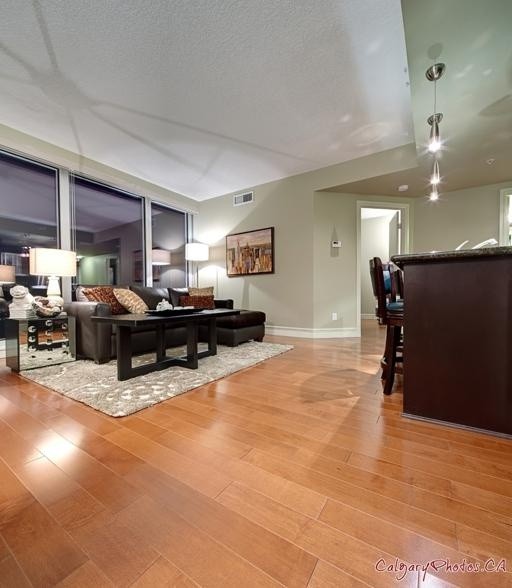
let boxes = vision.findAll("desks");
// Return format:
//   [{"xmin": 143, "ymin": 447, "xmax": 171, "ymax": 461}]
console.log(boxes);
[
  {"xmin": 391, "ymin": 246, "xmax": 512, "ymax": 441},
  {"xmin": 5, "ymin": 318, "xmax": 76, "ymax": 370},
  {"xmin": 90, "ymin": 308, "xmax": 240, "ymax": 381}
]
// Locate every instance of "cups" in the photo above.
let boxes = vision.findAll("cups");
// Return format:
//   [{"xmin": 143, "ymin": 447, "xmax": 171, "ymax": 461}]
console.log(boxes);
[{"xmin": 174, "ymin": 306, "xmax": 194, "ymax": 310}]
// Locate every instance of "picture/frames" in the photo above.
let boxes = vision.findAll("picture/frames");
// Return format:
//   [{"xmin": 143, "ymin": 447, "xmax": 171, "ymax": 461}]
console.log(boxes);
[
  {"xmin": 225, "ymin": 227, "xmax": 274, "ymax": 276},
  {"xmin": 133, "ymin": 246, "xmax": 160, "ymax": 282}
]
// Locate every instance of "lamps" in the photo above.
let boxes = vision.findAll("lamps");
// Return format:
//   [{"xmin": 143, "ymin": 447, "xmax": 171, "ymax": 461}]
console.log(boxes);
[
  {"xmin": 152, "ymin": 249, "xmax": 171, "ymax": 287},
  {"xmin": 29, "ymin": 247, "xmax": 77, "ymax": 308},
  {"xmin": 426, "ymin": 63, "xmax": 445, "ymax": 202},
  {"xmin": 185, "ymin": 242, "xmax": 209, "ymax": 288},
  {"xmin": 0, "ymin": 265, "xmax": 16, "ymax": 298}
]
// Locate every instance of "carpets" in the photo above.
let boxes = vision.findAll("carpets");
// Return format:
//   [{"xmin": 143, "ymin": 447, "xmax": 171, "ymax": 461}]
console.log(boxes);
[{"xmin": 18, "ymin": 339, "xmax": 295, "ymax": 417}]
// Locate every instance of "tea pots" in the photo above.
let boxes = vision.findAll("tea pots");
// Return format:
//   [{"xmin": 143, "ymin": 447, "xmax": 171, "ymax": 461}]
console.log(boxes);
[{"xmin": 156, "ymin": 298, "xmax": 173, "ymax": 311}]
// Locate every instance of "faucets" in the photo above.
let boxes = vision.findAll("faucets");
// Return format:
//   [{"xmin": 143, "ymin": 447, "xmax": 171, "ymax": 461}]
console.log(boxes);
[{"xmin": 472, "ymin": 238, "xmax": 498, "ymax": 250}]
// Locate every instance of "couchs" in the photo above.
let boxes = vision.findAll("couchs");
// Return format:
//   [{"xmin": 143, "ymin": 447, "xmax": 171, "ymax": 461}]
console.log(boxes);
[{"xmin": 64, "ymin": 285, "xmax": 266, "ymax": 365}]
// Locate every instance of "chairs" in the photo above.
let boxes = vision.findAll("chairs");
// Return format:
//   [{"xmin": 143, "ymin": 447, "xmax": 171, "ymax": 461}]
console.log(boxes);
[{"xmin": 370, "ymin": 256, "xmax": 404, "ymax": 395}]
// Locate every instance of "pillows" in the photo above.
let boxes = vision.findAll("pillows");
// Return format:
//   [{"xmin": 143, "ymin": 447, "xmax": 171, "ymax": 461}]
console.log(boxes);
[
  {"xmin": 188, "ymin": 286, "xmax": 215, "ymax": 308},
  {"xmin": 81, "ymin": 287, "xmax": 126, "ymax": 314},
  {"xmin": 178, "ymin": 295, "xmax": 214, "ymax": 308},
  {"xmin": 113, "ymin": 288, "xmax": 149, "ymax": 314}
]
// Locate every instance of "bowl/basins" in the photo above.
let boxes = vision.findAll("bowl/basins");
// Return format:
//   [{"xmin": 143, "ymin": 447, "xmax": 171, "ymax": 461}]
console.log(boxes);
[{"xmin": 30, "ymin": 296, "xmax": 63, "ymax": 318}]
